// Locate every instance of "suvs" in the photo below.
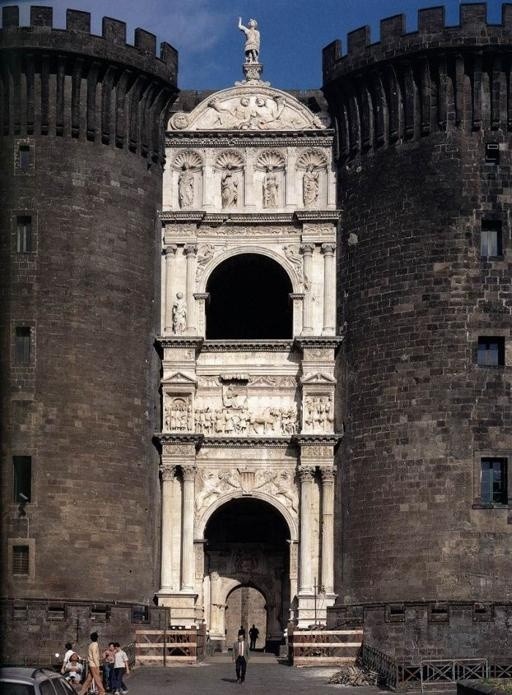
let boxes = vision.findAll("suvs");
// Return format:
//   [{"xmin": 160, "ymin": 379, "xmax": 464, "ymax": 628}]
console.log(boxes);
[{"xmin": 0, "ymin": 666, "xmax": 79, "ymax": 695}]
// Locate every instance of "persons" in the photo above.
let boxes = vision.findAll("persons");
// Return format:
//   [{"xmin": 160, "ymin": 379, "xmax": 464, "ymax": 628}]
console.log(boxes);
[
  {"xmin": 237, "ymin": 625, "xmax": 246, "ymax": 639},
  {"xmin": 249, "ymin": 624, "xmax": 259, "ymax": 650},
  {"xmin": 165, "ymin": 386, "xmax": 334, "ymax": 435},
  {"xmin": 302, "ymin": 163, "xmax": 320, "ymax": 208},
  {"xmin": 262, "ymin": 164, "xmax": 280, "ymax": 208},
  {"xmin": 232, "ymin": 633, "xmax": 251, "ymax": 690},
  {"xmin": 238, "ymin": 16, "xmax": 260, "ymax": 63},
  {"xmin": 221, "ymin": 162, "xmax": 239, "ymax": 208},
  {"xmin": 172, "ymin": 292, "xmax": 188, "ymax": 335},
  {"xmin": 60, "ymin": 632, "xmax": 129, "ymax": 695},
  {"xmin": 178, "ymin": 162, "xmax": 194, "ymax": 211}
]
